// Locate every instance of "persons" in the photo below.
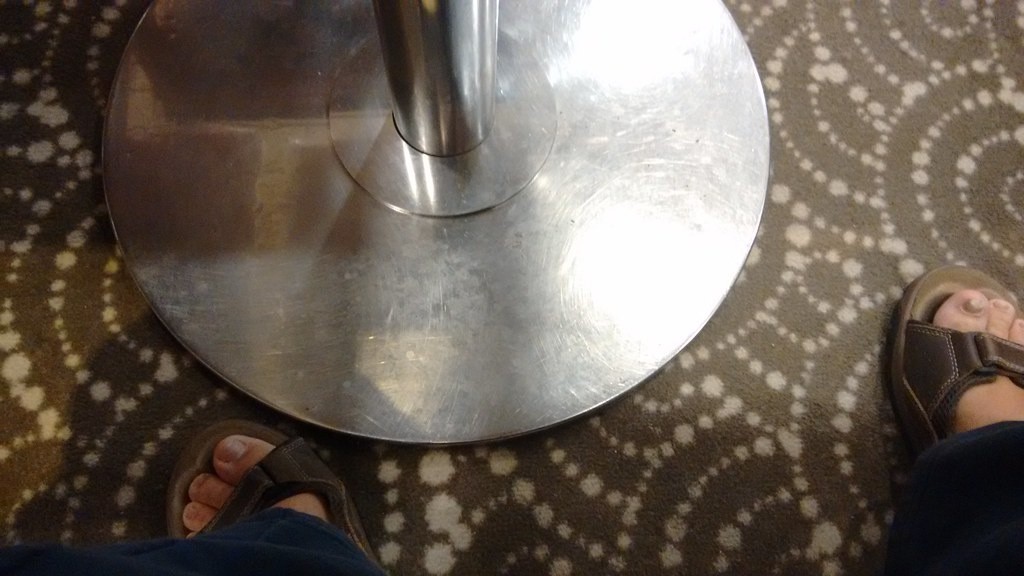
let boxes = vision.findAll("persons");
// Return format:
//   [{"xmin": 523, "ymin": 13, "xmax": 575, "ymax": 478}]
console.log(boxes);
[{"xmin": 0, "ymin": 266, "xmax": 1023, "ymax": 576}]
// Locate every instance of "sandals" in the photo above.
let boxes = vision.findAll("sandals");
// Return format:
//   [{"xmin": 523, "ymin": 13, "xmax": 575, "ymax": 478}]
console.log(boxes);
[
  {"xmin": 890, "ymin": 264, "xmax": 1024, "ymax": 457},
  {"xmin": 166, "ymin": 419, "xmax": 388, "ymax": 574}
]
